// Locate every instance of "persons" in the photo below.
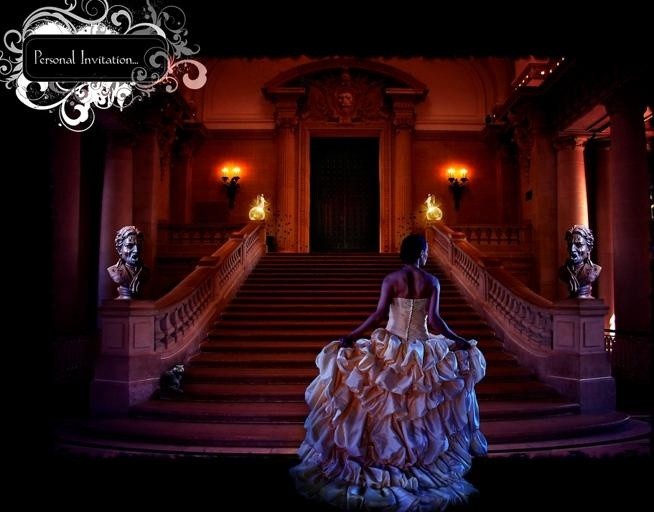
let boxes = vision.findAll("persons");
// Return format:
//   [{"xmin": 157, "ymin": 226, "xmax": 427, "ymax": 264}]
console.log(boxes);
[
  {"xmin": 557, "ymin": 225, "xmax": 602, "ymax": 291},
  {"xmin": 337, "ymin": 91, "xmax": 353, "ymax": 111},
  {"xmin": 107, "ymin": 226, "xmax": 147, "ymax": 296},
  {"xmin": 290, "ymin": 234, "xmax": 489, "ymax": 505}
]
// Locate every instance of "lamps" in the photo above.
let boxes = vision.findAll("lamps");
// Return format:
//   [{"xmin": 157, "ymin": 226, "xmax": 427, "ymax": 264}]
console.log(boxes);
[
  {"xmin": 424, "ymin": 193, "xmax": 443, "ymax": 220},
  {"xmin": 249, "ymin": 194, "xmax": 267, "ymax": 220},
  {"xmin": 448, "ymin": 168, "xmax": 468, "ymax": 190},
  {"xmin": 221, "ymin": 168, "xmax": 242, "ymax": 190}
]
